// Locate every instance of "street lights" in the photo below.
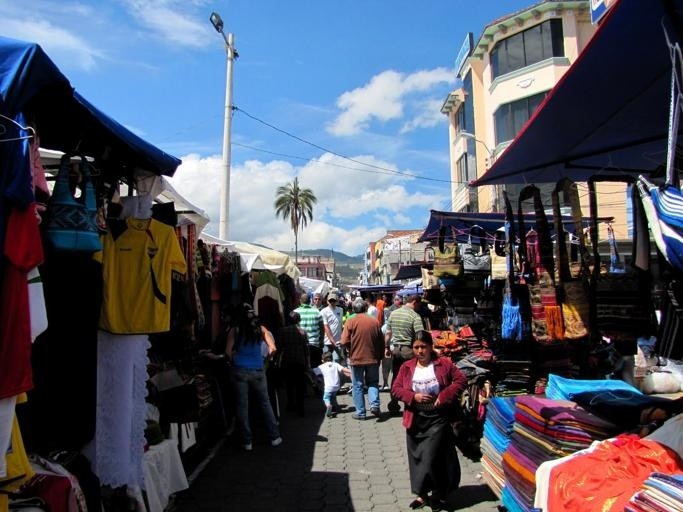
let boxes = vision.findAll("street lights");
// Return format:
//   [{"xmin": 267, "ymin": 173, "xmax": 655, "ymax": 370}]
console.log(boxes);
[
  {"xmin": 209, "ymin": 12, "xmax": 239, "ymax": 240},
  {"xmin": 459, "ymin": 132, "xmax": 497, "ymax": 210}
]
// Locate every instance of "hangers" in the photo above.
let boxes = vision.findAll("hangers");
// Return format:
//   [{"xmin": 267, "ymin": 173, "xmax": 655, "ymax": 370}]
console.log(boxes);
[
  {"xmin": 427, "ymin": 218, "xmax": 604, "ymax": 247},
  {"xmin": 0, "ymin": 108, "xmax": 104, "ymax": 180}
]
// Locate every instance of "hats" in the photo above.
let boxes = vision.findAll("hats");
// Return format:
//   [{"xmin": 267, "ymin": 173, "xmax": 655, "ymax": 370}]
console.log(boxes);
[{"xmin": 326, "ymin": 294, "xmax": 338, "ymax": 301}]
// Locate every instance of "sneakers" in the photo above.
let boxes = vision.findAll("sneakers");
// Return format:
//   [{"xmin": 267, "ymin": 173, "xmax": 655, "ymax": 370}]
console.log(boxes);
[
  {"xmin": 245, "ymin": 442, "xmax": 252, "ymax": 450},
  {"xmin": 326, "ymin": 404, "xmax": 332, "ymax": 417},
  {"xmin": 272, "ymin": 437, "xmax": 283, "ymax": 446},
  {"xmin": 351, "ymin": 413, "xmax": 367, "ymax": 420},
  {"xmin": 409, "ymin": 500, "xmax": 429, "ymax": 510},
  {"xmin": 370, "ymin": 406, "xmax": 382, "ymax": 418},
  {"xmin": 430, "ymin": 501, "xmax": 445, "ymax": 511}
]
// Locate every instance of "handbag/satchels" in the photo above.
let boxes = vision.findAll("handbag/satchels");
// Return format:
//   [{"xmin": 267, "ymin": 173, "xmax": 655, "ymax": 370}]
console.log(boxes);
[
  {"xmin": 503, "ymin": 284, "xmax": 527, "ymax": 341},
  {"xmin": 561, "ymin": 301, "xmax": 589, "ymax": 339},
  {"xmin": 526, "ymin": 263, "xmax": 558, "ymax": 306},
  {"xmin": 420, "ymin": 246, "xmax": 442, "ymax": 292},
  {"xmin": 490, "ymin": 228, "xmax": 520, "ymax": 281},
  {"xmin": 531, "ymin": 305, "xmax": 564, "ymax": 345},
  {"xmin": 42, "ymin": 152, "xmax": 105, "ymax": 253},
  {"xmin": 433, "ymin": 222, "xmax": 461, "ymax": 277},
  {"xmin": 463, "ymin": 224, "xmax": 491, "ymax": 275}
]
